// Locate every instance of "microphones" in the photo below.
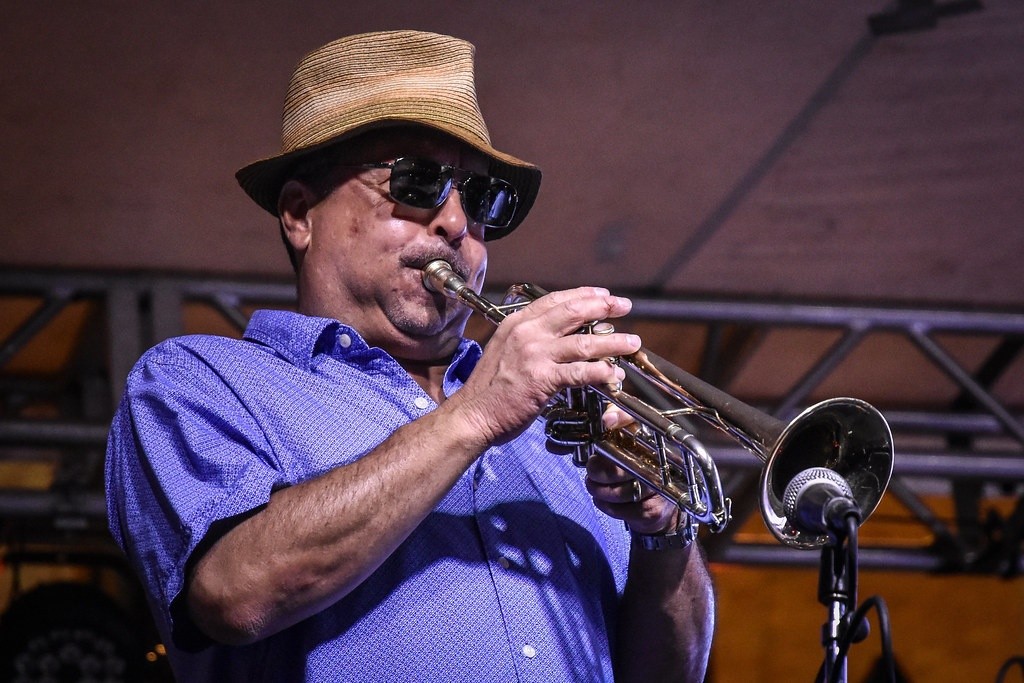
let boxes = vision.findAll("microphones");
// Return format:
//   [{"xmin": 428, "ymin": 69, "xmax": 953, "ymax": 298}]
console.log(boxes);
[{"xmin": 783, "ymin": 467, "xmax": 862, "ymax": 536}]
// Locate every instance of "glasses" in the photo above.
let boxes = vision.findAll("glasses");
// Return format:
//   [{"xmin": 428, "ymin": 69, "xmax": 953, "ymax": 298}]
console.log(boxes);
[{"xmin": 301, "ymin": 155, "xmax": 520, "ymax": 228}]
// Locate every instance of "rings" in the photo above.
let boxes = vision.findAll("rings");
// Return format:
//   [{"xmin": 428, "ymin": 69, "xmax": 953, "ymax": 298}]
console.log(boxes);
[{"xmin": 633, "ymin": 479, "xmax": 641, "ymax": 503}]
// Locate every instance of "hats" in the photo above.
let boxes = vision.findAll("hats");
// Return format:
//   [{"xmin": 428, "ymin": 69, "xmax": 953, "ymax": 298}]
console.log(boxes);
[{"xmin": 233, "ymin": 30, "xmax": 542, "ymax": 242}]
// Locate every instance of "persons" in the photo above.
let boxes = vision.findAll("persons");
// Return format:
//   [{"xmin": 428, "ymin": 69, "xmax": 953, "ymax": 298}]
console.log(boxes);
[{"xmin": 103, "ymin": 30, "xmax": 713, "ymax": 683}]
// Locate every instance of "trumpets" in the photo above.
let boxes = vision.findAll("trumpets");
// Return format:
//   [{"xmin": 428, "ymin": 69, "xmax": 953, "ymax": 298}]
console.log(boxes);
[{"xmin": 423, "ymin": 254, "xmax": 897, "ymax": 551}]
